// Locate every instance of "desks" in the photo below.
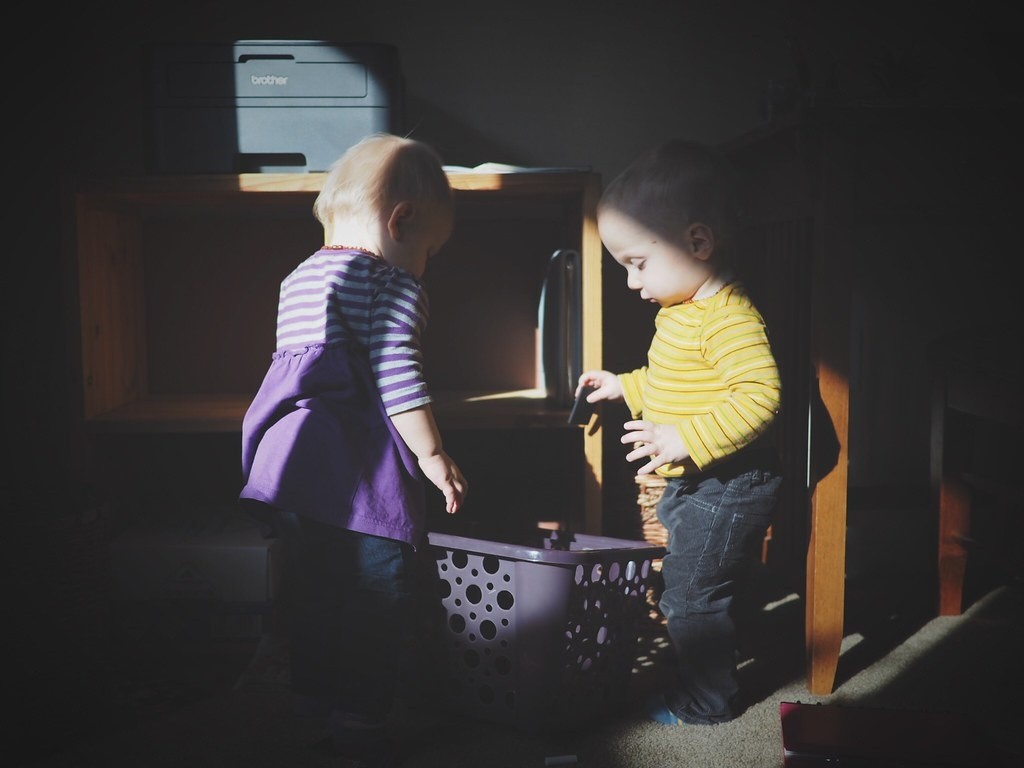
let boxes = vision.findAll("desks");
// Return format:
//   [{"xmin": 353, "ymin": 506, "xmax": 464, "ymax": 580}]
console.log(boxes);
[{"xmin": 714, "ymin": 96, "xmax": 1024, "ymax": 696}]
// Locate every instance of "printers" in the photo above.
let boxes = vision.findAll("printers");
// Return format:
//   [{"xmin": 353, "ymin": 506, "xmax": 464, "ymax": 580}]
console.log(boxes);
[{"xmin": 141, "ymin": 39, "xmax": 408, "ymax": 173}]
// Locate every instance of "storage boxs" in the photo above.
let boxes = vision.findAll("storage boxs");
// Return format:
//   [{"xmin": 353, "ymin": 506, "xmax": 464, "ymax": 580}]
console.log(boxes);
[
  {"xmin": 109, "ymin": 505, "xmax": 287, "ymax": 602},
  {"xmin": 418, "ymin": 524, "xmax": 668, "ymax": 734}
]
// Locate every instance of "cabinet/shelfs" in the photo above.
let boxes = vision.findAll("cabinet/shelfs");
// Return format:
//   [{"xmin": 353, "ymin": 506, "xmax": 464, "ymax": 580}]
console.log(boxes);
[{"xmin": 50, "ymin": 165, "xmax": 605, "ymax": 539}]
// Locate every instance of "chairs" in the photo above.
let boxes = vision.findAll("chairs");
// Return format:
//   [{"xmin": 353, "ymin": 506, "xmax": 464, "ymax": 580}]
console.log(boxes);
[{"xmin": 924, "ymin": 328, "xmax": 1024, "ymax": 615}]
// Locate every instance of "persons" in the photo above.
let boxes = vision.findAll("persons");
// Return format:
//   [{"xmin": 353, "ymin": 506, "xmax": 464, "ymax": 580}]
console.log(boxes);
[
  {"xmin": 243, "ymin": 132, "xmax": 468, "ymax": 762},
  {"xmin": 574, "ymin": 136, "xmax": 785, "ymax": 727}
]
